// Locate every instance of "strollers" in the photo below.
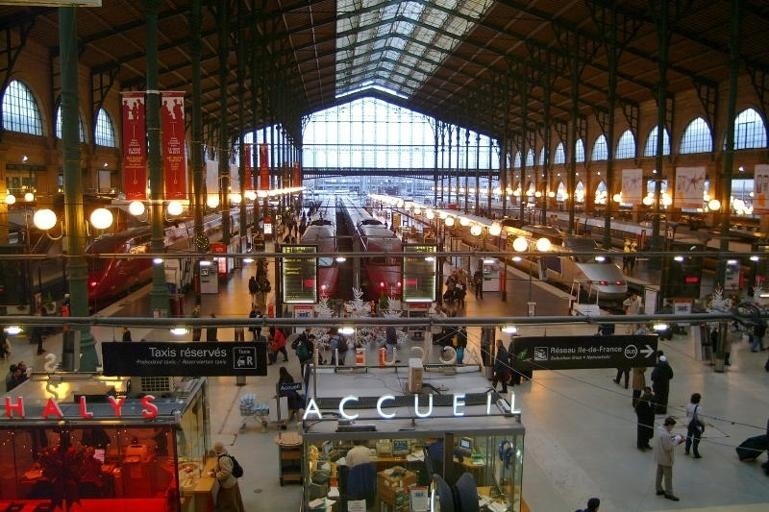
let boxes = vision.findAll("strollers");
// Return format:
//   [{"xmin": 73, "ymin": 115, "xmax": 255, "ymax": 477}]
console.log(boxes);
[{"xmin": 235, "ymin": 394, "xmax": 278, "ymax": 434}]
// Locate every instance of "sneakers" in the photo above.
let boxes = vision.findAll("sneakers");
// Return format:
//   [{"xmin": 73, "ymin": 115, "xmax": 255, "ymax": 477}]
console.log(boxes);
[
  {"xmin": 656, "ymin": 491, "xmax": 680, "ymax": 502},
  {"xmin": 684, "ymin": 450, "xmax": 702, "ymax": 458}
]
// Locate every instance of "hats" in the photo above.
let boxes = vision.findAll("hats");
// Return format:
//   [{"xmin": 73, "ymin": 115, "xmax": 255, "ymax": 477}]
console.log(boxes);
[{"xmin": 213, "ymin": 441, "xmax": 226, "ymax": 454}]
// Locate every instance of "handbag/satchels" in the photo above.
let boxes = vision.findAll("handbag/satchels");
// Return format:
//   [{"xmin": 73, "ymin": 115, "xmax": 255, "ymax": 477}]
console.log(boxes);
[
  {"xmin": 687, "ymin": 416, "xmax": 704, "ymax": 435},
  {"xmin": 295, "ymin": 345, "xmax": 310, "ymax": 363}
]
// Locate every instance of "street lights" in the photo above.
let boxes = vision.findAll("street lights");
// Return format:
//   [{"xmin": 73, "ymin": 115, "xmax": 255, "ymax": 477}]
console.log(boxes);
[{"xmin": 380, "ymin": 198, "xmax": 551, "ymax": 321}]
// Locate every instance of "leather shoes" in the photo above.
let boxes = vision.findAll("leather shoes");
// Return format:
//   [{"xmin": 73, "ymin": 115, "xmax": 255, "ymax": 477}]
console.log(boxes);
[
  {"xmin": 498, "ymin": 382, "xmax": 513, "ymax": 393},
  {"xmin": 637, "ymin": 444, "xmax": 652, "ymax": 451},
  {"xmin": 613, "ymin": 379, "xmax": 619, "ymax": 384}
]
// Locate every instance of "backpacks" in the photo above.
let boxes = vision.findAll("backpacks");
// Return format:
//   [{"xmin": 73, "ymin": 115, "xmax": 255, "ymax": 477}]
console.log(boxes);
[{"xmin": 217, "ymin": 454, "xmax": 243, "ymax": 478}]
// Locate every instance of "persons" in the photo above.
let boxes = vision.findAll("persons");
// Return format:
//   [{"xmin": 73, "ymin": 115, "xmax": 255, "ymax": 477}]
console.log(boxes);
[
  {"xmin": 611, "ymin": 367, "xmax": 630, "ymax": 389},
  {"xmin": 634, "ymin": 387, "xmax": 656, "ymax": 452},
  {"xmin": 624, "ymin": 292, "xmax": 642, "ymax": 332},
  {"xmin": 632, "ymin": 365, "xmax": 647, "ymax": 390},
  {"xmin": 61, "ymin": 300, "xmax": 71, "ymax": 332},
  {"xmin": 685, "ymin": 392, "xmax": 705, "ymax": 458},
  {"xmin": 629, "ymin": 238, "xmax": 639, "ymax": 270},
  {"xmin": 652, "ymin": 355, "xmax": 674, "ymax": 414},
  {"xmin": 121, "ymin": 328, "xmax": 134, "ymax": 343},
  {"xmin": 420, "ymin": 438, "xmax": 445, "ymax": 483},
  {"xmin": 208, "ymin": 442, "xmax": 245, "ymax": 512},
  {"xmin": 621, "ymin": 238, "xmax": 631, "ymax": 273},
  {"xmin": 0, "ymin": 305, "xmax": 48, "ymax": 392},
  {"xmin": 275, "ymin": 367, "xmax": 308, "ymax": 429},
  {"xmin": 574, "ymin": 497, "xmax": 600, "ymax": 512},
  {"xmin": 345, "ymin": 439, "xmax": 378, "ymax": 508},
  {"xmin": 188, "ymin": 264, "xmax": 488, "ymax": 371},
  {"xmin": 654, "ymin": 416, "xmax": 687, "ymax": 502},
  {"xmin": 750, "ymin": 322, "xmax": 767, "ymax": 353}
]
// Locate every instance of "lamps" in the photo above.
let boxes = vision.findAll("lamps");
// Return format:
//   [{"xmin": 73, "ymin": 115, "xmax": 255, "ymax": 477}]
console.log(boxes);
[
  {"xmin": 367, "ymin": 194, "xmax": 550, "ymax": 253},
  {"xmin": 7, "ymin": 184, "xmax": 307, "ymax": 242}
]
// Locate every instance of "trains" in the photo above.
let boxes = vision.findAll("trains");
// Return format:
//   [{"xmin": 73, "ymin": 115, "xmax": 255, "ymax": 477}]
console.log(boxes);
[
  {"xmin": 339, "ymin": 194, "xmax": 405, "ymax": 301},
  {"xmin": 365, "ymin": 192, "xmax": 629, "ymax": 303},
  {"xmin": 85, "ymin": 197, "xmax": 285, "ymax": 302},
  {"xmin": 297, "ymin": 195, "xmax": 337, "ymax": 303}
]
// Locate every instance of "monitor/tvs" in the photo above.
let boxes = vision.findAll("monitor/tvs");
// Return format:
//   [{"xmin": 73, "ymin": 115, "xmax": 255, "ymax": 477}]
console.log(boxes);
[
  {"xmin": 393, "ymin": 440, "xmax": 407, "ymax": 452},
  {"xmin": 460, "ymin": 440, "xmax": 470, "ymax": 447},
  {"xmin": 92, "ymin": 448, "xmax": 106, "ymax": 464}
]
